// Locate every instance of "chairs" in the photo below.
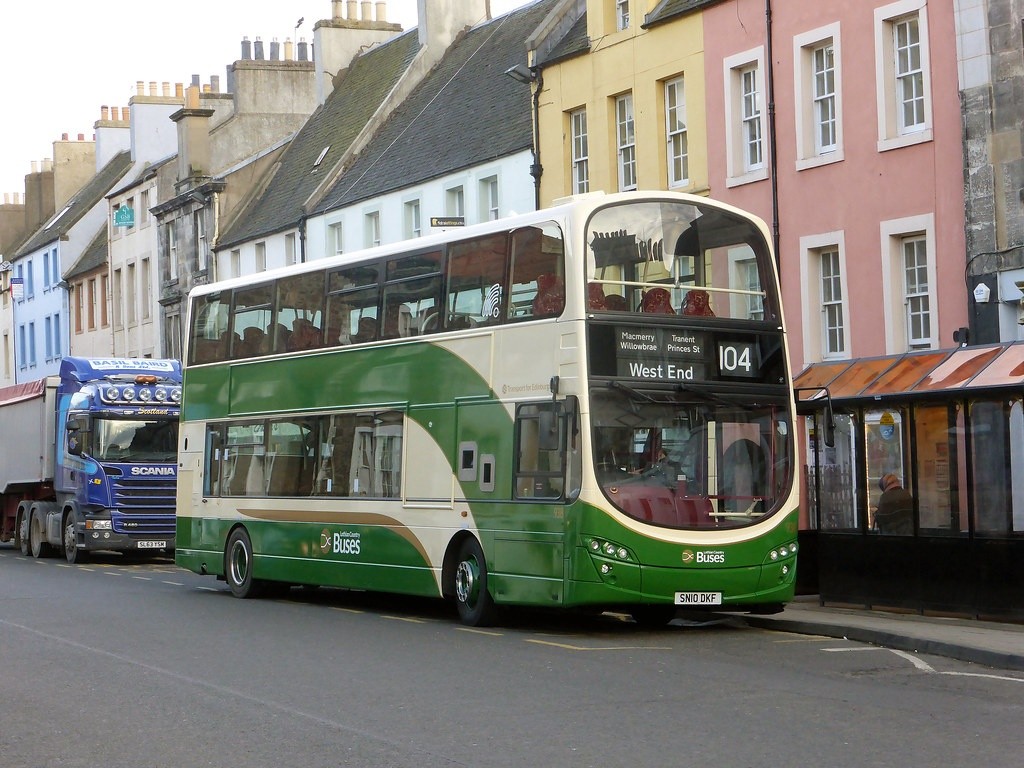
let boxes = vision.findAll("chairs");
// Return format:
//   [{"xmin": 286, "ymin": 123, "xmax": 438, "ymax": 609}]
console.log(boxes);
[
  {"xmin": 236, "ymin": 327, "xmax": 264, "ymax": 355},
  {"xmin": 588, "ymin": 278, "xmax": 608, "ymax": 310},
  {"xmin": 681, "ymin": 290, "xmax": 715, "ymax": 317},
  {"xmin": 532, "ymin": 272, "xmax": 564, "ymax": 316},
  {"xmin": 641, "ymin": 288, "xmax": 677, "ymax": 315},
  {"xmin": 605, "ymin": 294, "xmax": 625, "ymax": 310},
  {"xmin": 267, "ymin": 323, "xmax": 293, "ymax": 352},
  {"xmin": 215, "ymin": 331, "xmax": 242, "ymax": 359},
  {"xmin": 386, "ymin": 303, "xmax": 412, "ymax": 337},
  {"xmin": 357, "ymin": 316, "xmax": 378, "ymax": 339},
  {"xmin": 426, "ymin": 306, "xmax": 451, "ymax": 330},
  {"xmin": 286, "ymin": 319, "xmax": 321, "ymax": 350}
]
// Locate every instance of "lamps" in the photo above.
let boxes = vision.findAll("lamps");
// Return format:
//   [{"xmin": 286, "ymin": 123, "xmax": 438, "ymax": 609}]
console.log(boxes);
[
  {"xmin": 504, "ymin": 64, "xmax": 536, "ymax": 85},
  {"xmin": 57, "ymin": 281, "xmax": 71, "ymax": 291},
  {"xmin": 187, "ymin": 192, "xmax": 205, "ymax": 205}
]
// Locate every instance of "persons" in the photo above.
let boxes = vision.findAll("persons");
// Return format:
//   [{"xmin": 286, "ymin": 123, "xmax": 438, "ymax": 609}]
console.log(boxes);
[{"xmin": 871, "ymin": 474, "xmax": 914, "ymax": 535}]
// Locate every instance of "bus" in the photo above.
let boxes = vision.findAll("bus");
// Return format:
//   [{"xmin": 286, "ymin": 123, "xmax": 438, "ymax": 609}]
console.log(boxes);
[{"xmin": 173, "ymin": 191, "xmax": 835, "ymax": 633}]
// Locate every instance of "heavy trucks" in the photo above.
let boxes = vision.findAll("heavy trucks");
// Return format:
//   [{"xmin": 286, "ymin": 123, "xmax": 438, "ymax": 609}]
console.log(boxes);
[{"xmin": 0, "ymin": 358, "xmax": 180, "ymax": 565}]
